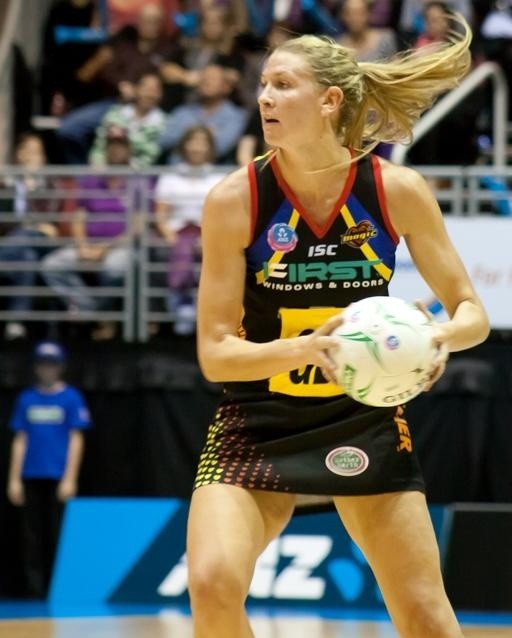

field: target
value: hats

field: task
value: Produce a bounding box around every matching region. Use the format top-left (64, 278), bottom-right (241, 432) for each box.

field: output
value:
top-left (106, 125), bottom-right (129, 141)
top-left (34, 342), bottom-right (64, 364)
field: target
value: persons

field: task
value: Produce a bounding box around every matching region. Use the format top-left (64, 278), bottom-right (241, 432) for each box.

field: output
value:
top-left (0, 1), bottom-right (511, 345)
top-left (6, 341), bottom-right (92, 601)
top-left (187, 11), bottom-right (493, 638)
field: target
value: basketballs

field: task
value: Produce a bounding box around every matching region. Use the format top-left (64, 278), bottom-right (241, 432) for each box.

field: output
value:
top-left (325, 296), bottom-right (440, 408)
